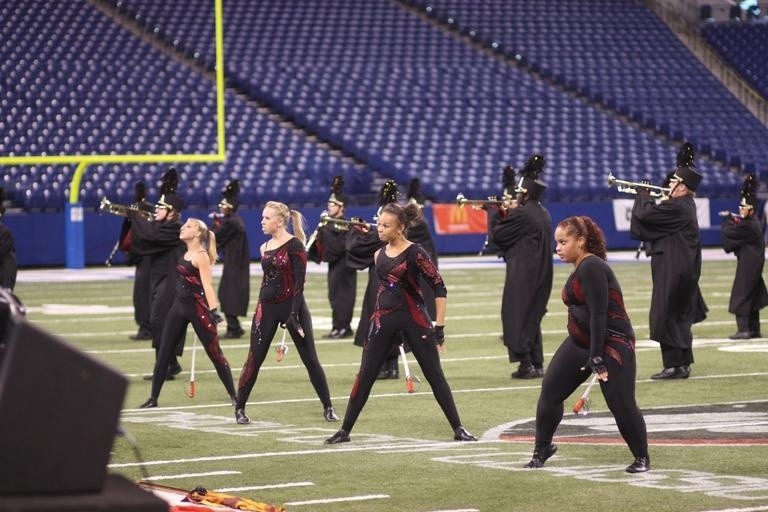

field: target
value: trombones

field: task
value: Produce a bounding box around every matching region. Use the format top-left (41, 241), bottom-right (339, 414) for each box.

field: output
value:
top-left (95, 195), bottom-right (157, 223)
top-left (605, 171), bottom-right (673, 196)
top-left (318, 208), bottom-right (377, 233)
top-left (454, 191), bottom-right (518, 210)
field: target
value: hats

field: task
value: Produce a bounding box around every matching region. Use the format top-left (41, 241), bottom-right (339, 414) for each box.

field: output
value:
top-left (501, 166), bottom-right (516, 201)
top-left (371, 180), bottom-right (400, 220)
top-left (327, 176), bottom-right (349, 218)
top-left (133, 179), bottom-right (155, 215)
top-left (737, 173), bottom-right (757, 217)
top-left (218, 179), bottom-right (241, 217)
top-left (153, 166), bottom-right (184, 222)
top-left (669, 142), bottom-right (703, 195)
top-left (405, 177), bottom-right (426, 209)
top-left (514, 153), bottom-right (548, 205)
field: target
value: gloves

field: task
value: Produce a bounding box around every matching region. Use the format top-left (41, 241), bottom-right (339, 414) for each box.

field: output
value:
top-left (432, 326), bottom-right (445, 345)
top-left (287, 312), bottom-right (302, 330)
top-left (585, 356), bottom-right (607, 375)
top-left (209, 308), bottom-right (223, 325)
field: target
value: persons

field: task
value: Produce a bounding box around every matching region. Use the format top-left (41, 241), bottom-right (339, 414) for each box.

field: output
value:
top-left (1, 206), bottom-right (24, 346)
top-left (324, 202), bottom-right (478, 445)
top-left (239, 202), bottom-right (338, 423)
top-left (126, 194), bottom-right (189, 340)
top-left (525, 217), bottom-right (650, 473)
top-left (139, 218), bottom-right (237, 407)
top-left (631, 167), bottom-right (709, 380)
top-left (306, 193), bottom-right (358, 341)
top-left (722, 196), bottom-right (767, 338)
top-left (484, 179), bottom-right (553, 379)
top-left (119, 199), bottom-right (160, 338)
top-left (345, 196), bottom-right (437, 381)
top-left (210, 193), bottom-right (249, 340)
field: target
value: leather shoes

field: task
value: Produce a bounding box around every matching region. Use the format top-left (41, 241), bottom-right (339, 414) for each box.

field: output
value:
top-left (332, 327), bottom-right (355, 338)
top-left (524, 444), bottom-right (559, 469)
top-left (229, 393), bottom-right (237, 406)
top-left (128, 326), bottom-right (151, 340)
top-left (511, 364), bottom-right (538, 379)
top-left (626, 455), bottom-right (651, 474)
top-left (323, 407), bottom-right (340, 422)
top-left (143, 374), bottom-right (175, 381)
top-left (650, 366), bottom-right (687, 380)
top-left (728, 331), bottom-right (752, 339)
top-left (535, 368), bottom-right (544, 377)
top-left (139, 398), bottom-right (158, 408)
top-left (453, 426), bottom-right (477, 442)
top-left (375, 367), bottom-right (401, 379)
top-left (166, 359), bottom-right (182, 375)
top-left (682, 366), bottom-right (691, 379)
top-left (220, 329), bottom-right (241, 339)
top-left (324, 328), bottom-right (338, 339)
top-left (324, 429), bottom-right (350, 444)
top-left (240, 327), bottom-right (245, 335)
top-left (389, 368), bottom-right (398, 379)
top-left (235, 408), bottom-right (251, 424)
top-left (752, 330), bottom-right (761, 338)
